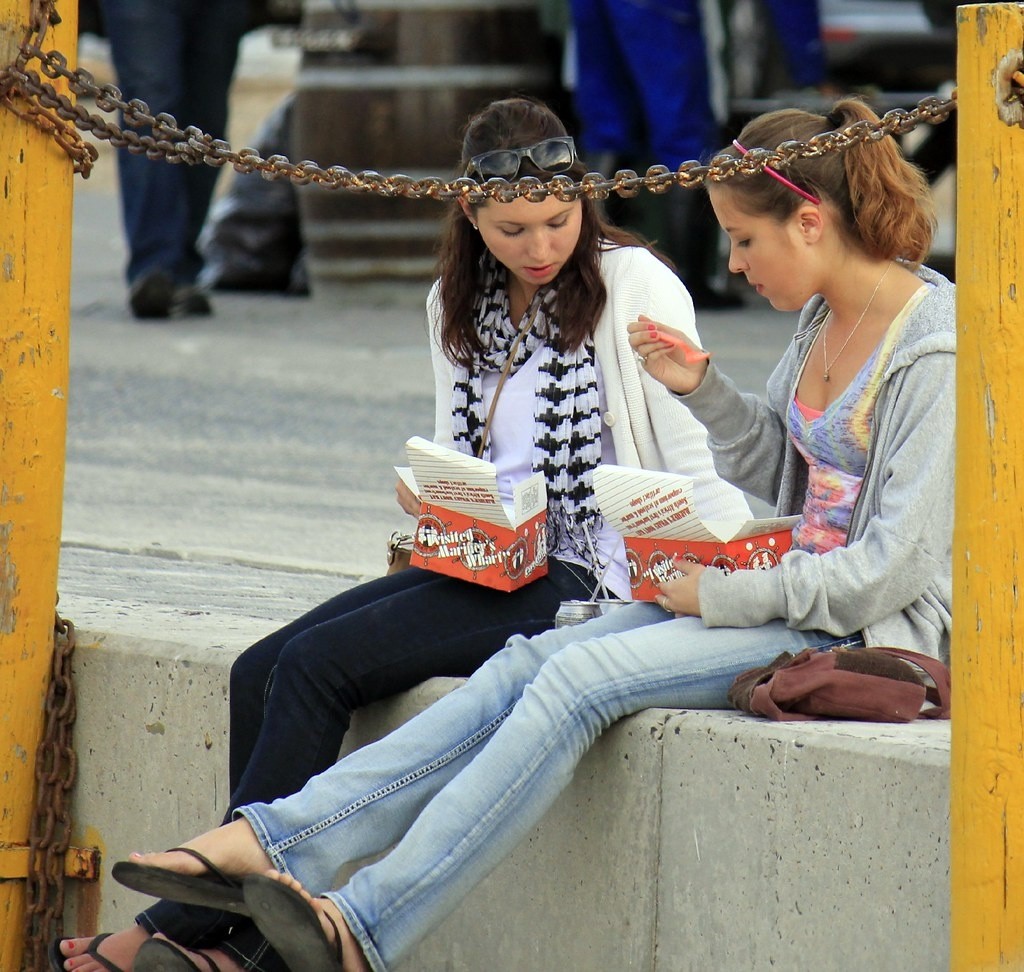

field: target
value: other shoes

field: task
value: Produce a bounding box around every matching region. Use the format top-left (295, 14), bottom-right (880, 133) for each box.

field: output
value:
top-left (130, 260), bottom-right (180, 319)
top-left (172, 282), bottom-right (215, 320)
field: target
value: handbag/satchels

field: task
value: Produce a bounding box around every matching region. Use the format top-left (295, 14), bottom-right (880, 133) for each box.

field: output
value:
top-left (732, 633), bottom-right (926, 727)
top-left (381, 528), bottom-right (413, 582)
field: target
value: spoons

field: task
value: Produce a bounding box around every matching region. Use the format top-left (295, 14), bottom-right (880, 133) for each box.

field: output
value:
top-left (657, 332), bottom-right (715, 363)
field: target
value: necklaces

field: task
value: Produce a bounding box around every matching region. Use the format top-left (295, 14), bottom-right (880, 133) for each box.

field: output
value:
top-left (823, 260), bottom-right (895, 382)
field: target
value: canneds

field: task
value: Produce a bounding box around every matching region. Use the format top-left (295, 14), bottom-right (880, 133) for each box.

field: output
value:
top-left (555, 600), bottom-right (602, 629)
top-left (595, 599), bottom-right (633, 615)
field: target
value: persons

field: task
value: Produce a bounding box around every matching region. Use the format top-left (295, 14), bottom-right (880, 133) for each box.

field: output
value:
top-left (561, 0), bottom-right (847, 313)
top-left (77, 0), bottom-right (305, 317)
top-left (111, 95), bottom-right (957, 971)
top-left (44, 98), bottom-right (721, 972)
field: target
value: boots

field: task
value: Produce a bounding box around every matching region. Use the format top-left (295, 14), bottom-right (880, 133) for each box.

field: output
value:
top-left (580, 143), bottom-right (639, 238)
top-left (660, 174), bottom-right (750, 316)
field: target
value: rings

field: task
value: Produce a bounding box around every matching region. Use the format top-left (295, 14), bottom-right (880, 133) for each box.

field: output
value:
top-left (661, 596), bottom-right (673, 615)
top-left (635, 350), bottom-right (648, 365)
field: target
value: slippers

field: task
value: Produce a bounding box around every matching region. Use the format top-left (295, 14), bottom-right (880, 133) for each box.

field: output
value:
top-left (241, 869), bottom-right (355, 972)
top-left (128, 931), bottom-right (224, 972)
top-left (110, 848), bottom-right (252, 924)
top-left (48, 928), bottom-right (122, 972)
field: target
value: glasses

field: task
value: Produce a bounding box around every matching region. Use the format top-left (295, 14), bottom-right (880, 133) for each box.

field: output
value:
top-left (461, 136), bottom-right (581, 189)
